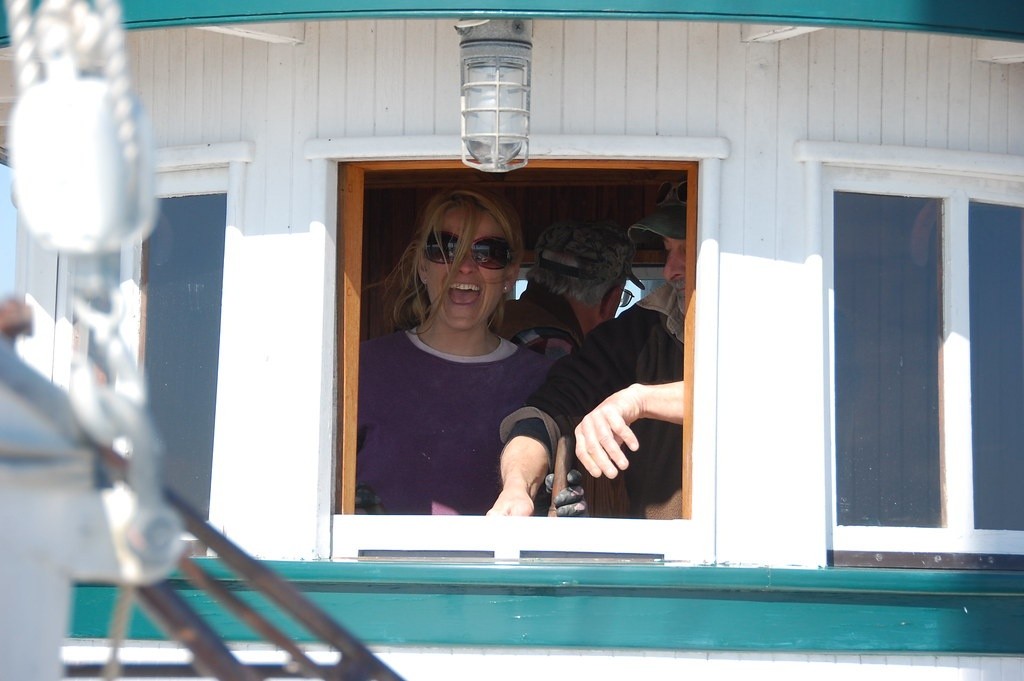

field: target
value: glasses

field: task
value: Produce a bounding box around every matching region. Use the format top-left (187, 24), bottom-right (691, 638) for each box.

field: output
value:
top-left (423, 231), bottom-right (512, 270)
top-left (656, 181), bottom-right (687, 206)
top-left (616, 287), bottom-right (635, 307)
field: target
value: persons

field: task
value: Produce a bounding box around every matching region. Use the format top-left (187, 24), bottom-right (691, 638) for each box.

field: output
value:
top-left (354, 188), bottom-right (588, 517)
top-left (487, 218), bottom-right (645, 518)
top-left (485, 180), bottom-right (687, 519)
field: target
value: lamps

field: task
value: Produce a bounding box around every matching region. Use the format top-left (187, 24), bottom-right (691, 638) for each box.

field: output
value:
top-left (456, 17), bottom-right (530, 174)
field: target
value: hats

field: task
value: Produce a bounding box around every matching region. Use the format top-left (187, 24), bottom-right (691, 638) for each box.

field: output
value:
top-left (533, 221), bottom-right (646, 290)
top-left (628, 171), bottom-right (687, 240)
